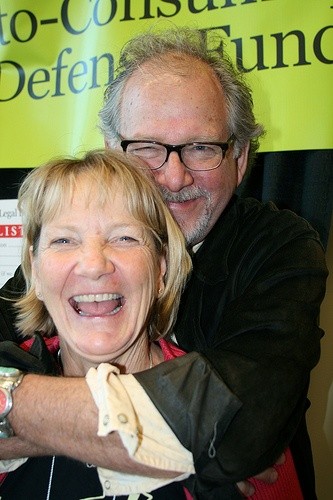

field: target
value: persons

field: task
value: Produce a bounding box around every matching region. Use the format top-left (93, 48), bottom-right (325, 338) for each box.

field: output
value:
top-left (1, 23), bottom-right (332, 500)
top-left (0, 152), bottom-right (287, 500)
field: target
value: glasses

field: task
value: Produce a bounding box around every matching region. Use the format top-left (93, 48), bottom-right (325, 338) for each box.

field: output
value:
top-left (115, 132), bottom-right (235, 171)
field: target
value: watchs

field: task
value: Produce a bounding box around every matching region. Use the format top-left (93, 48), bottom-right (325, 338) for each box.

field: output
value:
top-left (0, 363), bottom-right (24, 440)
